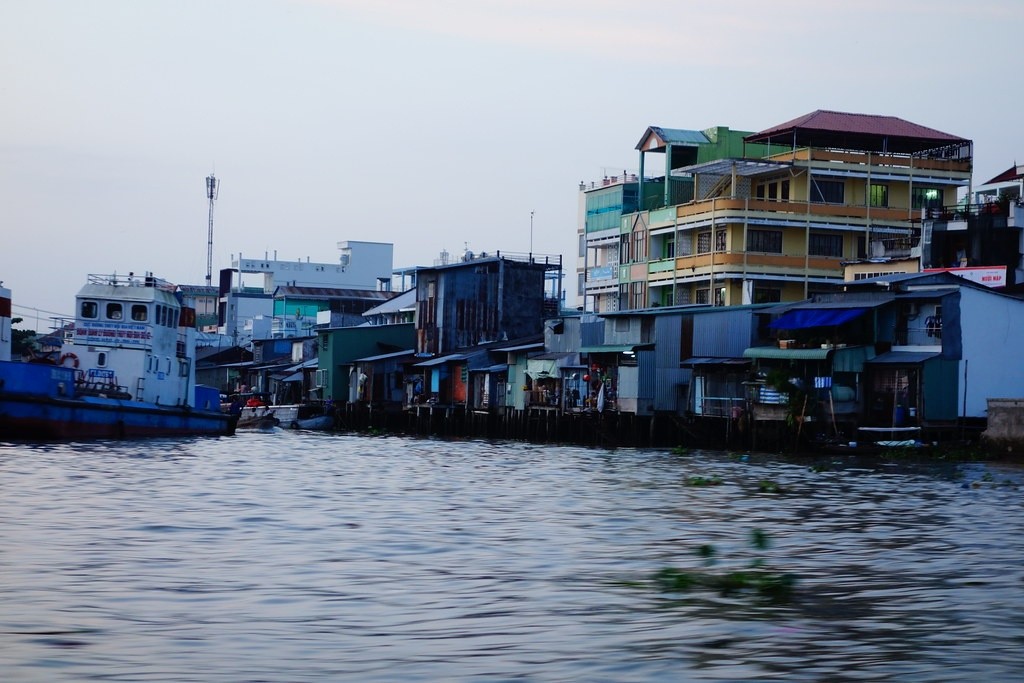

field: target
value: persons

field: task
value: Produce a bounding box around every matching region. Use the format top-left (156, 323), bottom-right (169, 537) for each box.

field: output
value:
top-left (128, 272), bottom-right (136, 287)
top-left (325, 395), bottom-right (333, 414)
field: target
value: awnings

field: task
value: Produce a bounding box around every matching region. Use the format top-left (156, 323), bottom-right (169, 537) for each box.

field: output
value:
top-left (865, 351), bottom-right (940, 363)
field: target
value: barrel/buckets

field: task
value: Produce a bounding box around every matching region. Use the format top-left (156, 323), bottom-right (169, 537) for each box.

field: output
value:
top-left (895, 406), bottom-right (904, 425)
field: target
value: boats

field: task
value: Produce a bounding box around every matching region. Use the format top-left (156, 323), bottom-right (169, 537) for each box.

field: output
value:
top-left (0, 264), bottom-right (252, 437)
top-left (240, 401), bottom-right (299, 433)
top-left (295, 413), bottom-right (336, 431)
top-left (807, 436), bottom-right (940, 463)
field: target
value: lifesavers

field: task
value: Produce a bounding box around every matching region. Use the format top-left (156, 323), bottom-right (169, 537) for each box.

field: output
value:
top-left (290, 421), bottom-right (300, 430)
top-left (60, 352), bottom-right (80, 369)
